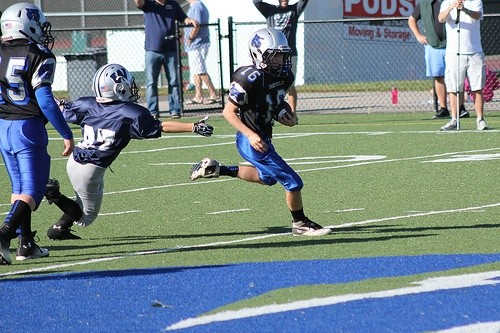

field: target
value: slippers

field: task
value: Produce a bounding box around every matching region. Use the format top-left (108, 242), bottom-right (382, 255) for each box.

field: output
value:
top-left (207, 97), bottom-right (220, 104)
top-left (185, 99), bottom-right (203, 105)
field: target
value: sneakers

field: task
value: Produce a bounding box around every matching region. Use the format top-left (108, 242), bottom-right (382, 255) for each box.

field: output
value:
top-left (432, 107), bottom-right (450, 118)
top-left (42, 178), bottom-right (60, 204)
top-left (292, 217), bottom-right (332, 236)
top-left (189, 157), bottom-right (222, 180)
top-left (440, 120), bottom-right (460, 131)
top-left (47, 226), bottom-right (81, 240)
top-left (459, 105), bottom-right (468, 117)
top-left (477, 119), bottom-right (488, 130)
top-left (16, 230), bottom-right (49, 260)
top-left (0, 222), bottom-right (19, 265)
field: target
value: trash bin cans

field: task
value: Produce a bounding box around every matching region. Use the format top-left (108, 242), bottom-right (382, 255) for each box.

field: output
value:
top-left (62, 50), bottom-right (108, 102)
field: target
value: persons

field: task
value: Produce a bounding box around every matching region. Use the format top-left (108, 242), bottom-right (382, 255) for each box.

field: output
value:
top-left (408, 0), bottom-right (470, 119)
top-left (0, 1), bottom-right (74, 261)
top-left (188, 27), bottom-right (333, 238)
top-left (440, 0), bottom-right (489, 131)
top-left (134, 0), bottom-right (199, 120)
top-left (43, 63), bottom-right (213, 240)
top-left (253, 0), bottom-right (309, 125)
top-left (184, 0), bottom-right (222, 105)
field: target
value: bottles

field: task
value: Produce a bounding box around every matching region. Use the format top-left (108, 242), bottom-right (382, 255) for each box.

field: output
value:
top-left (392, 87), bottom-right (398, 104)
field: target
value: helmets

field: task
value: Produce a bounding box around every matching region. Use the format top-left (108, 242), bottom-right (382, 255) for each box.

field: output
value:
top-left (92, 63), bottom-right (136, 103)
top-left (0, 2), bottom-right (47, 43)
top-left (248, 28), bottom-right (290, 69)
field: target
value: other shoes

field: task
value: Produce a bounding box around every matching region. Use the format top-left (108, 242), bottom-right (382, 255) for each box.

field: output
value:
top-left (153, 115), bottom-right (160, 118)
top-left (172, 115), bottom-right (181, 119)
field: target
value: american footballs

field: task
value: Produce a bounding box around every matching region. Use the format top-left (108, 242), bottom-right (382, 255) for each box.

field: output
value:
top-left (273, 100), bottom-right (292, 122)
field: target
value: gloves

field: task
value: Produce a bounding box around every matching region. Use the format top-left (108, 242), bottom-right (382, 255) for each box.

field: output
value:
top-left (192, 115), bottom-right (214, 137)
